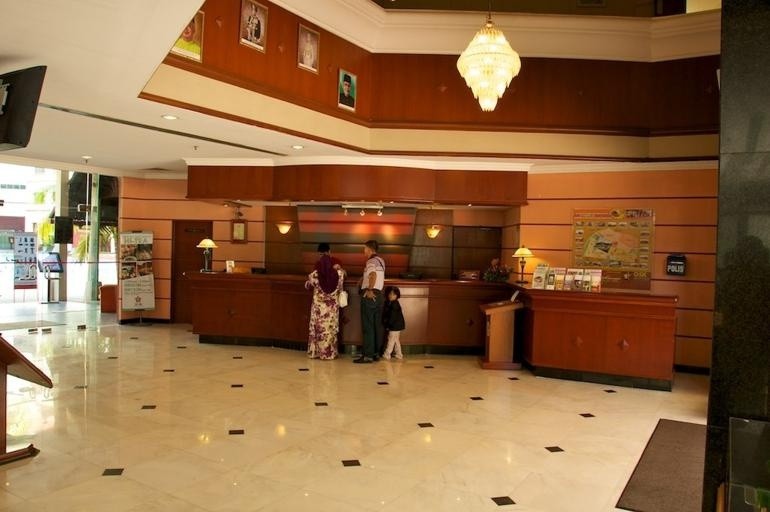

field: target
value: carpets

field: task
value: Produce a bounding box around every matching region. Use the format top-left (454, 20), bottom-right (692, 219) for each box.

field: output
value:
top-left (614, 417), bottom-right (707, 512)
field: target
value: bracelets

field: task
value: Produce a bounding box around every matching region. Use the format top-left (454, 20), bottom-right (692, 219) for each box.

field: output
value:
top-left (338, 266), bottom-right (342, 270)
top-left (367, 288), bottom-right (373, 291)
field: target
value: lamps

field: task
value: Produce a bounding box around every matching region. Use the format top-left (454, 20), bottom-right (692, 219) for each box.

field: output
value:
top-left (511, 245), bottom-right (536, 284)
top-left (455, 1), bottom-right (523, 114)
top-left (196, 236), bottom-right (218, 272)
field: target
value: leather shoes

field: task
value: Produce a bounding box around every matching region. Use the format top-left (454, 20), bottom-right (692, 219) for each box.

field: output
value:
top-left (353, 355), bottom-right (373, 363)
top-left (373, 353), bottom-right (380, 361)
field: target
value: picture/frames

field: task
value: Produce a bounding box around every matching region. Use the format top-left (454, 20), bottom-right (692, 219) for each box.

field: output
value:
top-left (230, 219), bottom-right (248, 244)
top-left (168, 10), bottom-right (205, 65)
top-left (297, 22), bottom-right (321, 76)
top-left (239, 0), bottom-right (269, 55)
top-left (338, 67), bottom-right (358, 114)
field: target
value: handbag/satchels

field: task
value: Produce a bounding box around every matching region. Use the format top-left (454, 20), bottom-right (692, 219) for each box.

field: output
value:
top-left (337, 290), bottom-right (348, 308)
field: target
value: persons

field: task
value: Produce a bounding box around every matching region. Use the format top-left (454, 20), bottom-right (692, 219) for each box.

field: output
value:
top-left (379, 286), bottom-right (406, 359)
top-left (311, 242), bottom-right (347, 278)
top-left (353, 240), bottom-right (386, 363)
top-left (304, 255), bottom-right (344, 361)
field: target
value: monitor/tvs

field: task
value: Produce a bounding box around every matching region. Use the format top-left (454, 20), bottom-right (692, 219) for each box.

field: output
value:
top-left (0, 65), bottom-right (47, 152)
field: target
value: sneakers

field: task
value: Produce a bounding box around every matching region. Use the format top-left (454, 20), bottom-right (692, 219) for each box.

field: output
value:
top-left (392, 355), bottom-right (402, 360)
